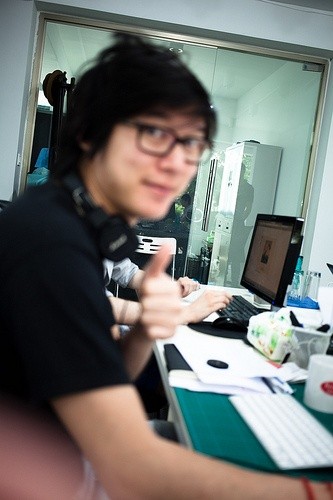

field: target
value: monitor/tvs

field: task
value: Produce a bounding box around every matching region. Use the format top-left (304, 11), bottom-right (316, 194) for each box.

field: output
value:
top-left (240, 214), bottom-right (305, 308)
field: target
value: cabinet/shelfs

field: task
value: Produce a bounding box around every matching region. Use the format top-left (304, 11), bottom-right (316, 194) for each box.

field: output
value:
top-left (207, 142), bottom-right (283, 286)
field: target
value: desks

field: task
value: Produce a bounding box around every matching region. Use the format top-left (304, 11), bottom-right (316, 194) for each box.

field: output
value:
top-left (154, 284), bottom-right (333, 479)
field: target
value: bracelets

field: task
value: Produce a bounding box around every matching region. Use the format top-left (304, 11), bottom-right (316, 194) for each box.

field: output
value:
top-left (301, 477), bottom-right (315, 500)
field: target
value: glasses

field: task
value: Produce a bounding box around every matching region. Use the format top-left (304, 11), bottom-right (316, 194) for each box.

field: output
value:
top-left (119, 119), bottom-right (214, 166)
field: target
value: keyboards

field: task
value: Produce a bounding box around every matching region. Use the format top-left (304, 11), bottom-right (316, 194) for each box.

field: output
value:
top-left (216, 295), bottom-right (261, 325)
top-left (228, 393), bottom-right (333, 470)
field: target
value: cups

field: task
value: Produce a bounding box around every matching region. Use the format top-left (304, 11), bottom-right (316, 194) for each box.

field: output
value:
top-left (284, 325), bottom-right (331, 384)
top-left (304, 354), bottom-right (333, 414)
top-left (286, 268), bottom-right (321, 309)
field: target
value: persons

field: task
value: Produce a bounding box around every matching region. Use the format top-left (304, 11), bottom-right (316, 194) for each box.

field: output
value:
top-left (0, 32), bottom-right (333, 500)
top-left (103, 254), bottom-right (233, 326)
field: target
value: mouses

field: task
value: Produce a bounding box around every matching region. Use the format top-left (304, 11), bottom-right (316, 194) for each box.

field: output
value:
top-left (212, 316), bottom-right (239, 330)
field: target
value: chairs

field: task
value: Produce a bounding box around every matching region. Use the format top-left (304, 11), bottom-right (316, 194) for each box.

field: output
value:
top-left (116, 235), bottom-right (178, 297)
